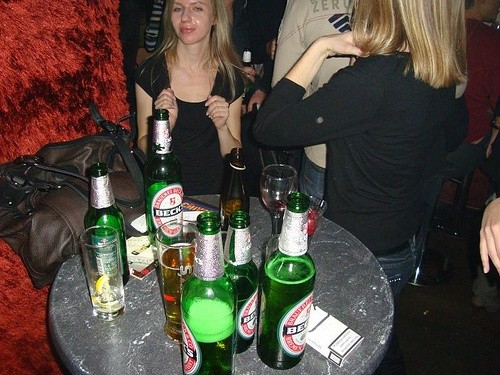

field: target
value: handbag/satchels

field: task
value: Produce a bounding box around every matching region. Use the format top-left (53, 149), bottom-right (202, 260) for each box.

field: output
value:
top-left (0, 101), bottom-right (154, 288)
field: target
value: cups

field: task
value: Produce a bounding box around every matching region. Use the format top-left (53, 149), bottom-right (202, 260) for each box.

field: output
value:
top-left (266, 235), bottom-right (279, 260)
top-left (308, 195), bottom-right (328, 244)
top-left (155, 220), bottom-right (201, 343)
top-left (79, 225), bottom-right (125, 322)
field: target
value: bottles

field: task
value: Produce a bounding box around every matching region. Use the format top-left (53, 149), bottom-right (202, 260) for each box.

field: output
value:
top-left (219, 147), bottom-right (250, 231)
top-left (222, 210), bottom-right (260, 354)
top-left (83, 163), bottom-right (129, 285)
top-left (145, 107), bottom-right (185, 244)
top-left (256, 192), bottom-right (317, 369)
top-left (241, 47), bottom-right (254, 94)
top-left (180, 212), bottom-right (238, 375)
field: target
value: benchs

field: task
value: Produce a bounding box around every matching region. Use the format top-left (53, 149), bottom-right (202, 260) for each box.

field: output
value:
top-left (0, 0), bottom-right (132, 375)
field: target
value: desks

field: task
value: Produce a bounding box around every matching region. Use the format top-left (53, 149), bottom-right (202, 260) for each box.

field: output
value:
top-left (45, 193), bottom-right (396, 375)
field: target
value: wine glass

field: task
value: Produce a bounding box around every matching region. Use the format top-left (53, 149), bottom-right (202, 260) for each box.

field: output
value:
top-left (260, 164), bottom-right (299, 235)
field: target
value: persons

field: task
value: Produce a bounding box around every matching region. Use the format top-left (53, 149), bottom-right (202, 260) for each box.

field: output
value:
top-left (460, 0), bottom-right (500, 275)
top-left (134, 0), bottom-right (288, 196)
top-left (271, 0), bottom-right (356, 205)
top-left (253, 0), bottom-right (465, 301)
